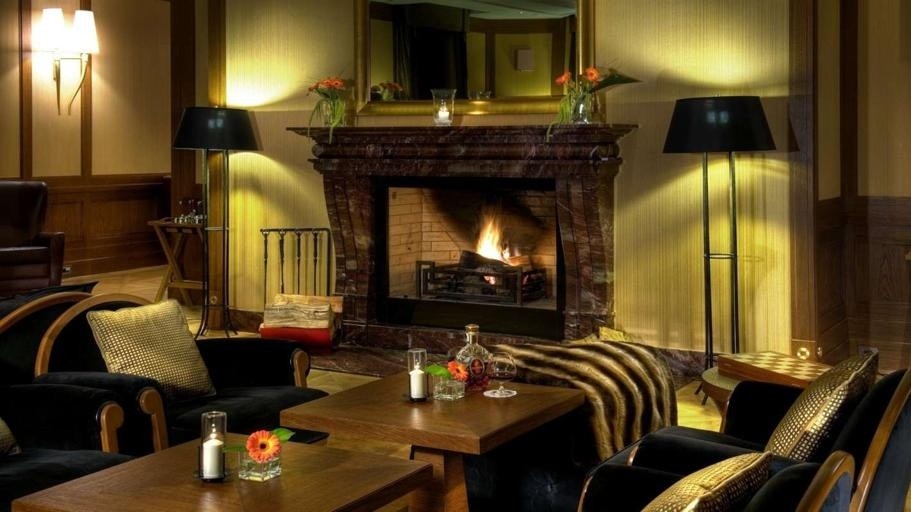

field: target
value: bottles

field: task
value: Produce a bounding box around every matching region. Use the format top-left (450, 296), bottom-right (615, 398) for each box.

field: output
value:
top-left (455, 322), bottom-right (494, 394)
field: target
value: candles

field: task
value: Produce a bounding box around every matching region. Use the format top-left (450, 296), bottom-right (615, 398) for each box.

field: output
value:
top-left (408, 357), bottom-right (428, 400)
top-left (431, 100), bottom-right (455, 126)
top-left (199, 420), bottom-right (227, 479)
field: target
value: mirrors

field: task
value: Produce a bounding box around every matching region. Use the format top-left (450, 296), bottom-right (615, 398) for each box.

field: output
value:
top-left (353, 1), bottom-right (593, 113)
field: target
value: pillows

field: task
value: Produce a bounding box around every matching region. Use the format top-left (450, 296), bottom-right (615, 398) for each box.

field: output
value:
top-left (84, 297), bottom-right (220, 400)
top-left (764, 344), bottom-right (882, 466)
top-left (0, 411), bottom-right (25, 463)
top-left (638, 446), bottom-right (775, 511)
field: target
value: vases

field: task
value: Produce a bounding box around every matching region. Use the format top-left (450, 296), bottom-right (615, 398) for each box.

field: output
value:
top-left (319, 100), bottom-right (348, 129)
top-left (562, 89), bottom-right (597, 126)
top-left (236, 448), bottom-right (288, 485)
top-left (432, 378), bottom-right (467, 401)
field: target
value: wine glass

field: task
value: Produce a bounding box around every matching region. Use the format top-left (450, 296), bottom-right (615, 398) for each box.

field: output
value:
top-left (487, 351), bottom-right (516, 395)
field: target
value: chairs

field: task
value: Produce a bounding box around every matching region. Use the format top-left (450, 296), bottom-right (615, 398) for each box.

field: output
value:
top-left (0, 289), bottom-right (95, 378)
top-left (34, 291), bottom-right (335, 458)
top-left (1, 177), bottom-right (69, 295)
top-left (0, 378), bottom-right (135, 512)
top-left (650, 365), bottom-right (911, 512)
top-left (578, 448), bottom-right (864, 512)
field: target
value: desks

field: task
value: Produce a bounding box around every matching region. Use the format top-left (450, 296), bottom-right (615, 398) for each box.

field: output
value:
top-left (277, 363), bottom-right (590, 511)
top-left (8, 428), bottom-right (437, 512)
top-left (150, 210), bottom-right (211, 309)
top-left (700, 365), bottom-right (744, 415)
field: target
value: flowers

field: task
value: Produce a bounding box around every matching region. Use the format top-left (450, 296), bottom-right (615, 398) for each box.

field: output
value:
top-left (309, 75), bottom-right (351, 129)
top-left (554, 64), bottom-right (604, 93)
top-left (245, 425), bottom-right (297, 464)
top-left (367, 77), bottom-right (404, 106)
top-left (427, 359), bottom-right (470, 381)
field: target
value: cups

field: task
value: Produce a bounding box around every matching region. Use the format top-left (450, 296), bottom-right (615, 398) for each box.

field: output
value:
top-left (202, 409), bottom-right (226, 480)
top-left (409, 347), bottom-right (428, 401)
top-left (430, 87), bottom-right (456, 126)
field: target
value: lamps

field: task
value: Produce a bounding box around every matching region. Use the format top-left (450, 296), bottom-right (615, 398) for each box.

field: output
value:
top-left (659, 94), bottom-right (779, 407)
top-left (169, 105), bottom-right (264, 341)
top-left (31, 2), bottom-right (103, 118)
